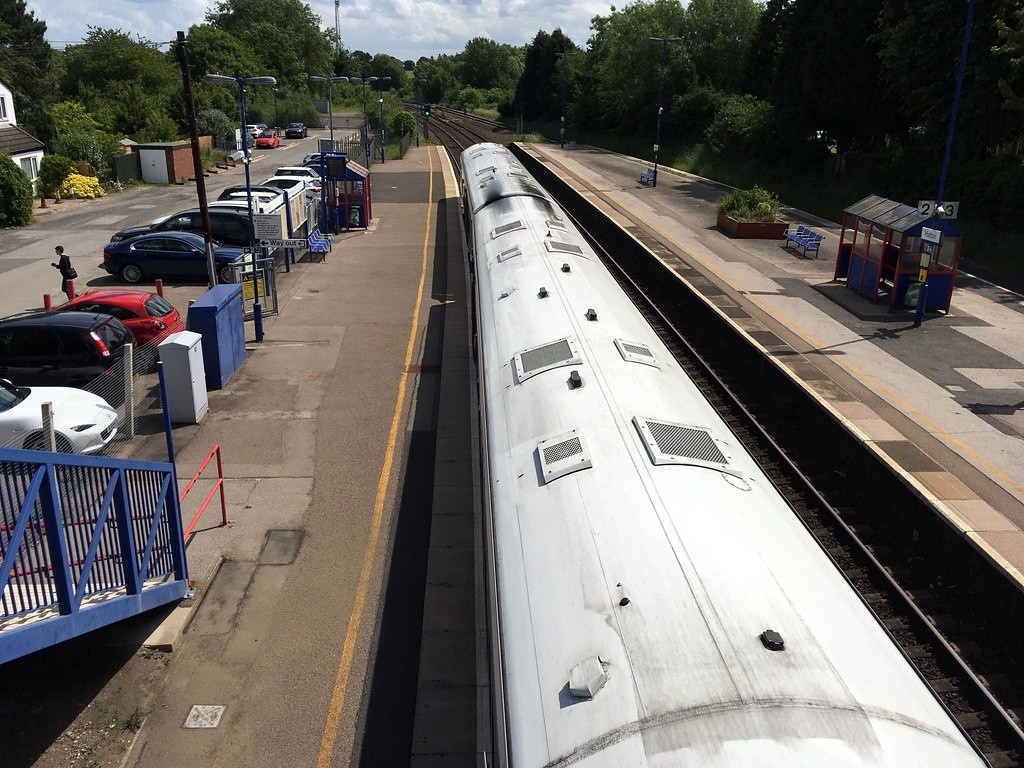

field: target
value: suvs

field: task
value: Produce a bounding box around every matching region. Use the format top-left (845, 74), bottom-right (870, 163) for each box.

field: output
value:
top-left (0, 311), bottom-right (138, 407)
top-left (274, 165), bottom-right (321, 183)
top-left (110, 205), bottom-right (260, 249)
top-left (246, 125), bottom-right (263, 138)
top-left (209, 185), bottom-right (285, 210)
top-left (254, 175), bottom-right (322, 202)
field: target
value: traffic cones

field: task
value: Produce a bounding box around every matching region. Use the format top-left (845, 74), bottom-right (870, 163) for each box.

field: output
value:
top-left (66, 184), bottom-right (77, 200)
top-left (38, 192), bottom-right (49, 208)
top-left (54, 188), bottom-right (64, 203)
top-left (114, 177), bottom-right (122, 191)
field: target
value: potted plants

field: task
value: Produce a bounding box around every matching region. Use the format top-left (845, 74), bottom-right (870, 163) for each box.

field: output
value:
top-left (715, 183), bottom-right (789, 240)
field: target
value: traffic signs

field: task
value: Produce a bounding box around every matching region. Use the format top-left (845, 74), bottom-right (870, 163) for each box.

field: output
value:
top-left (261, 240), bottom-right (306, 248)
top-left (244, 246), bottom-right (261, 253)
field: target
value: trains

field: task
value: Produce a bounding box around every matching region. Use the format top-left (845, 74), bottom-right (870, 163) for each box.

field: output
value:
top-left (459, 143), bottom-right (990, 768)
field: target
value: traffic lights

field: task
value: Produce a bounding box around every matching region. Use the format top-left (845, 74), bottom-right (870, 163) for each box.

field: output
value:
top-left (424, 104), bottom-right (431, 116)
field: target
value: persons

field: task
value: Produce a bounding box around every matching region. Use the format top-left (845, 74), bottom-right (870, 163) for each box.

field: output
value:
top-left (53, 246), bottom-right (79, 300)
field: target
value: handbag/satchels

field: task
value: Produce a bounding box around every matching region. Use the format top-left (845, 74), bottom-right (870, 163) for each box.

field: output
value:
top-left (63, 268), bottom-right (78, 279)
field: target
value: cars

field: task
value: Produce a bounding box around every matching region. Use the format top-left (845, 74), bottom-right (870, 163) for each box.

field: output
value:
top-left (0, 379), bottom-right (119, 454)
top-left (256, 132), bottom-right (280, 149)
top-left (295, 153), bottom-right (329, 176)
top-left (50, 289), bottom-right (185, 373)
top-left (285, 123), bottom-right (307, 139)
top-left (102, 231), bottom-right (245, 284)
top-left (247, 133), bottom-right (255, 149)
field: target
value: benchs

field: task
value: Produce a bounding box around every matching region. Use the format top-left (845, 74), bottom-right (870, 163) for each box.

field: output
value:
top-left (640, 168), bottom-right (653, 184)
top-left (783, 225), bottom-right (826, 258)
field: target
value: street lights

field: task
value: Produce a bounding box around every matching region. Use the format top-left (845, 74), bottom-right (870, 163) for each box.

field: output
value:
top-left (350, 77), bottom-right (377, 170)
top-left (377, 77), bottom-right (391, 122)
top-left (648, 36), bottom-right (682, 185)
top-left (206, 73), bottom-right (277, 342)
top-left (309, 76), bottom-right (349, 234)
top-left (555, 52), bottom-right (575, 149)
